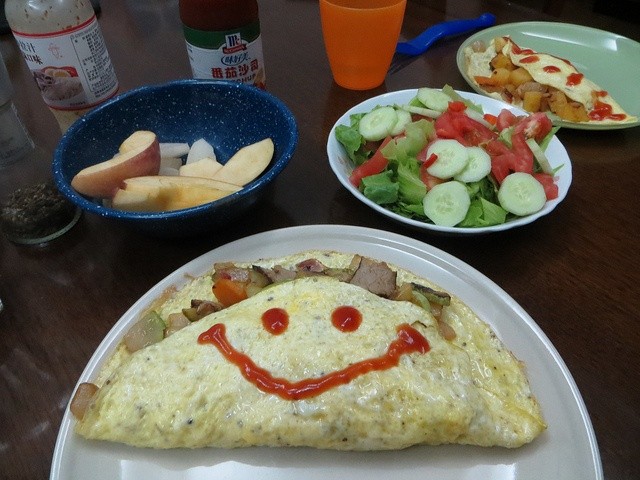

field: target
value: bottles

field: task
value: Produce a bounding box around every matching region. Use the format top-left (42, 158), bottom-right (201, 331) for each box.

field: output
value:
top-left (178, 0), bottom-right (267, 91)
top-left (5, 0), bottom-right (121, 137)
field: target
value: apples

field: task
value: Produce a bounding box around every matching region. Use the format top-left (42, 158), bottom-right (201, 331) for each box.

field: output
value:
top-left (111, 174), bottom-right (246, 212)
top-left (70, 129), bottom-right (161, 199)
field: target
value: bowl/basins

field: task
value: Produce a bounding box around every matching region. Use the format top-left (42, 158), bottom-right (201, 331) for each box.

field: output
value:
top-left (50, 78), bottom-right (299, 235)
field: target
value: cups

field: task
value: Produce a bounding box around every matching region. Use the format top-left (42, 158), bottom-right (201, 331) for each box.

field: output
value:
top-left (319, 1), bottom-right (407, 91)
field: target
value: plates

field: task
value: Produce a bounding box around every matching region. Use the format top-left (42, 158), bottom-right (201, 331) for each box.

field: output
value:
top-left (47, 223), bottom-right (605, 480)
top-left (327, 87), bottom-right (574, 234)
top-left (455, 20), bottom-right (640, 131)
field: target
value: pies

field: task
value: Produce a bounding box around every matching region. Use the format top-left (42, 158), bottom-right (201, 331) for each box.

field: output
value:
top-left (463, 32), bottom-right (638, 127)
top-left (67, 249), bottom-right (547, 453)
